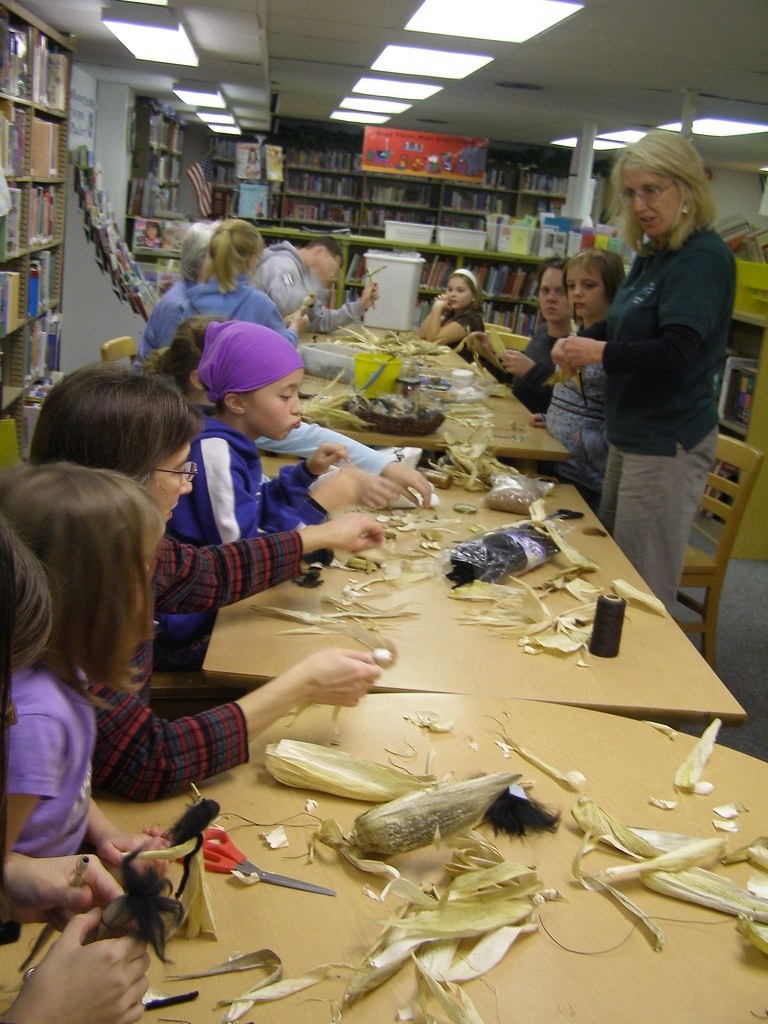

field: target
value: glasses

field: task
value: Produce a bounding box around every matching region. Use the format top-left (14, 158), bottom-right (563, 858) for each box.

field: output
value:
top-left (618, 182), bottom-right (675, 206)
top-left (154, 461), bottom-right (198, 481)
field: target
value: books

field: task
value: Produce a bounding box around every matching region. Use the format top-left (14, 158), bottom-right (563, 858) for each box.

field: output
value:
top-left (0, 28), bottom-right (67, 466)
top-left (73, 144), bottom-right (161, 321)
top-left (713, 212), bottom-right (768, 264)
top-left (126, 113), bottom-right (186, 218)
top-left (615, 254), bottom-right (621, 261)
top-left (486, 213), bottom-right (599, 251)
top-left (281, 148), bottom-right (566, 230)
top-left (416, 255), bottom-right (545, 337)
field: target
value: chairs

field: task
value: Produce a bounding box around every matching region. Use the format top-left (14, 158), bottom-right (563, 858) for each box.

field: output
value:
top-left (675, 432), bottom-right (766, 670)
top-left (98, 335), bottom-right (137, 368)
top-left (483, 322), bottom-right (513, 334)
top-left (485, 331), bottom-right (532, 354)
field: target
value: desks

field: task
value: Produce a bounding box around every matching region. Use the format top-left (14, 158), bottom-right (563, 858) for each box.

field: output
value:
top-left (201, 456), bottom-right (747, 727)
top-left (295, 324), bottom-right (569, 477)
top-left (1, 693), bottom-right (767, 1024)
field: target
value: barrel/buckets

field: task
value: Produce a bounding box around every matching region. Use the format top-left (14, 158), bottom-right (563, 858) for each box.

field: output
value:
top-left (354, 353), bottom-right (402, 395)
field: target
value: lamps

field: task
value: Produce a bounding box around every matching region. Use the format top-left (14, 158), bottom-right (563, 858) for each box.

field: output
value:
top-left (171, 83), bottom-right (242, 136)
top-left (99, 5), bottom-right (201, 69)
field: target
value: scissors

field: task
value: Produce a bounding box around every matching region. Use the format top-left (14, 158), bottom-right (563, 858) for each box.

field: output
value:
top-left (549, 509), bottom-right (584, 521)
top-left (201, 829), bottom-right (337, 899)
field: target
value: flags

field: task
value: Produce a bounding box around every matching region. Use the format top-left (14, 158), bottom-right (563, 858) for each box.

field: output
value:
top-left (188, 144), bottom-right (220, 217)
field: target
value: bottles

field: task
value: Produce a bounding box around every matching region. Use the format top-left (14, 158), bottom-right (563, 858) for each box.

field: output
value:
top-left (396, 377), bottom-right (420, 402)
top-left (401, 357), bottom-right (419, 377)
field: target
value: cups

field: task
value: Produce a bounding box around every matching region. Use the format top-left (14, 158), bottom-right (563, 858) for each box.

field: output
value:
top-left (452, 370), bottom-right (472, 389)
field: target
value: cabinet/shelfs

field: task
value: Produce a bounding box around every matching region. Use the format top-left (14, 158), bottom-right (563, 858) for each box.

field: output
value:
top-left (0, 1), bottom-right (80, 471)
top-left (128, 95), bottom-right (768, 559)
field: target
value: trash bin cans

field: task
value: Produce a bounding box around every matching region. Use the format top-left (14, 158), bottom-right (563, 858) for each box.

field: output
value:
top-left (362, 253), bottom-right (426, 331)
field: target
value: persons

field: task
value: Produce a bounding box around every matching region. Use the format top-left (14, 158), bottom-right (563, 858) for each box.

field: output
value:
top-left (138, 222), bottom-right (161, 249)
top-left (135, 219), bottom-right (380, 373)
top-left (550, 131), bottom-right (737, 617)
top-left (467, 249), bottom-right (625, 513)
top-left (0, 466), bottom-right (166, 1024)
top-left (243, 147), bottom-right (260, 180)
top-left (420, 267), bottom-right (484, 365)
top-left (30, 313), bottom-right (432, 802)
